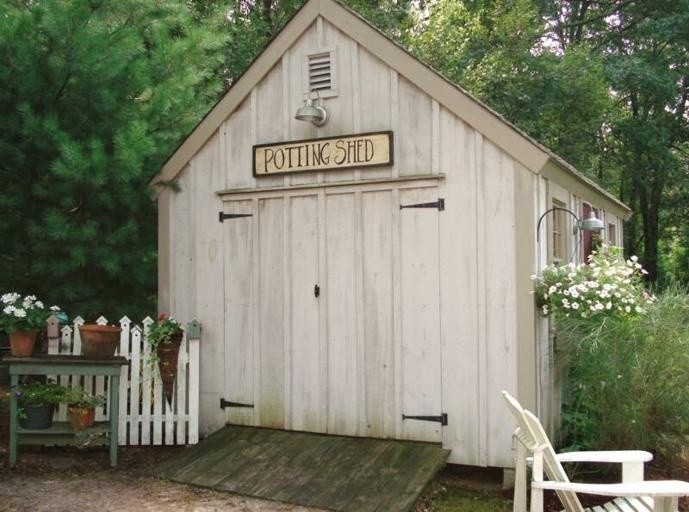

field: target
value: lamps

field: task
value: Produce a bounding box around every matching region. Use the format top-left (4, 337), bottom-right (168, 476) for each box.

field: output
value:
top-left (295, 90), bottom-right (330, 127)
top-left (576, 199), bottom-right (604, 237)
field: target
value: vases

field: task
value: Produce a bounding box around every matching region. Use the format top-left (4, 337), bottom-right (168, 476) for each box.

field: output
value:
top-left (78, 326), bottom-right (118, 360)
top-left (7, 330), bottom-right (36, 358)
top-left (20, 401), bottom-right (95, 429)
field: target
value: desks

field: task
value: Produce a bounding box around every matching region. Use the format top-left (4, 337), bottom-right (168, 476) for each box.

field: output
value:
top-left (3, 355), bottom-right (127, 466)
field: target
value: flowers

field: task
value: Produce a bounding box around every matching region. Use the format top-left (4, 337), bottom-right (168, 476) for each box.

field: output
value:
top-left (1, 292), bottom-right (50, 333)
top-left (145, 312), bottom-right (182, 364)
top-left (9, 377), bottom-right (107, 420)
top-left (532, 241), bottom-right (656, 355)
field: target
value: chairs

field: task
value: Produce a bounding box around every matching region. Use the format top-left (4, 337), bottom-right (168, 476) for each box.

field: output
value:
top-left (496, 388), bottom-right (688, 512)
